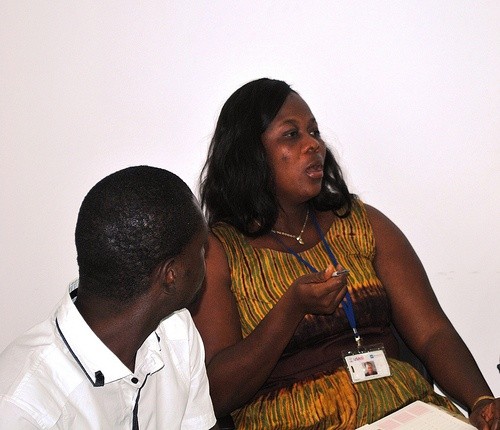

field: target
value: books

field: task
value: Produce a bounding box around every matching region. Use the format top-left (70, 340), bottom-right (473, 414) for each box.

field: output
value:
top-left (356, 401), bottom-right (478, 430)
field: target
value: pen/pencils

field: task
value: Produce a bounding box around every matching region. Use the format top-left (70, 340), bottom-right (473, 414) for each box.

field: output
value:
top-left (332, 269), bottom-right (350, 277)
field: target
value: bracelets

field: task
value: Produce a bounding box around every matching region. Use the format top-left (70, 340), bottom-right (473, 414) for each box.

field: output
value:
top-left (471, 396), bottom-right (495, 411)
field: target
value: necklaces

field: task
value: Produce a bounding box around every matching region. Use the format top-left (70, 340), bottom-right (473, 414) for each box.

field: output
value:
top-left (249, 208), bottom-right (310, 245)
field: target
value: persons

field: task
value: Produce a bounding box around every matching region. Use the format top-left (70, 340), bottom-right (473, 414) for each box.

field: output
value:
top-left (186, 78), bottom-right (500, 430)
top-left (0, 165), bottom-right (217, 430)
top-left (364, 362), bottom-right (377, 376)
